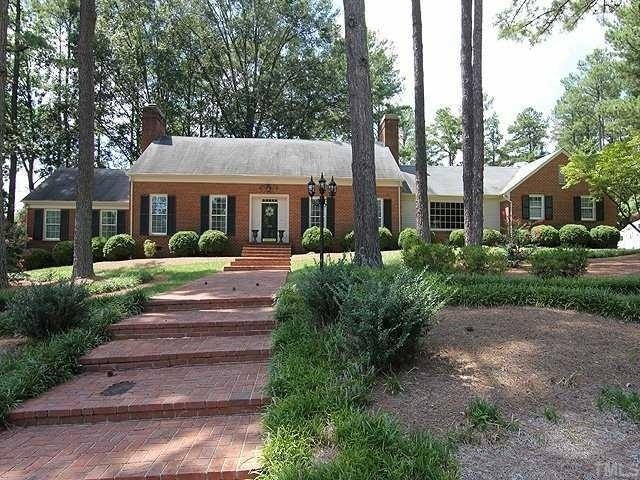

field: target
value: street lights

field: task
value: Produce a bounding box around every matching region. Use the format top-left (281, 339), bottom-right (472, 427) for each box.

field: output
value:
top-left (308, 170), bottom-right (337, 274)
